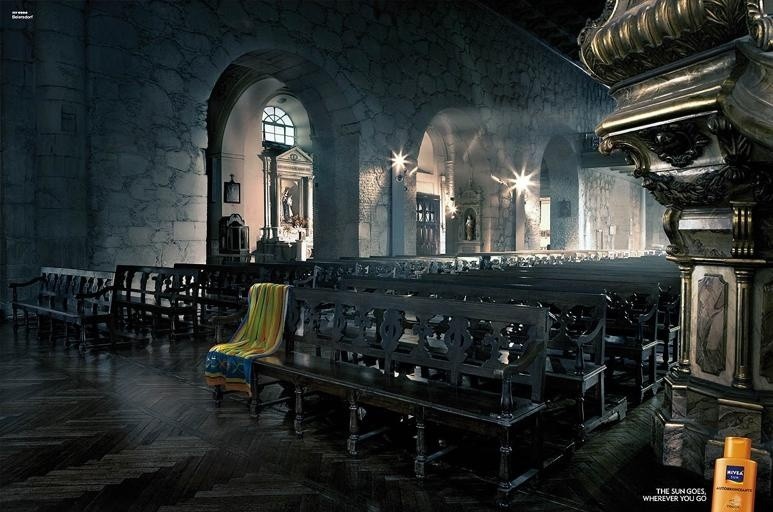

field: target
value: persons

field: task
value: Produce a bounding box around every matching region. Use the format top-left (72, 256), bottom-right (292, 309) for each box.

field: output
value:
top-left (282, 189), bottom-right (290, 220)
top-left (285, 194), bottom-right (293, 217)
top-left (464, 214), bottom-right (473, 242)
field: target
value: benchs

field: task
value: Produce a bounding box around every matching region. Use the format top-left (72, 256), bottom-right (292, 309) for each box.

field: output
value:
top-left (9, 256), bottom-right (681, 512)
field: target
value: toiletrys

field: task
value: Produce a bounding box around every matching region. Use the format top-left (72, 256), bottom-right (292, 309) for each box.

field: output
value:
top-left (711, 435), bottom-right (758, 512)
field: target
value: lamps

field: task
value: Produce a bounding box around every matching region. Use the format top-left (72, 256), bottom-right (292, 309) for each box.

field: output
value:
top-left (395, 166), bottom-right (406, 190)
